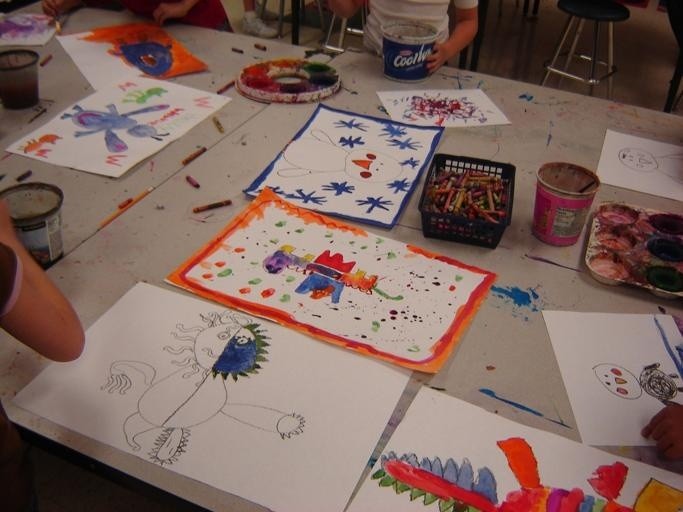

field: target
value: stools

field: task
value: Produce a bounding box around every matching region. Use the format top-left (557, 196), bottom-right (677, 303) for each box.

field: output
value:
top-left (540, 0), bottom-right (630, 101)
top-left (324, 0), bottom-right (368, 55)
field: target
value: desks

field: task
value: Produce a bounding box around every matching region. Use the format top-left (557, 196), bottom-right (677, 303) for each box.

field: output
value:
top-left (0, 0), bottom-right (334, 269)
top-left (1, 49), bottom-right (682, 512)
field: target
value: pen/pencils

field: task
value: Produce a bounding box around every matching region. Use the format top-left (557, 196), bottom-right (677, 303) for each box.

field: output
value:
top-left (182, 147), bottom-right (206, 165)
top-left (28, 109), bottom-right (47, 124)
top-left (16, 169), bottom-right (32, 181)
top-left (217, 80), bottom-right (235, 94)
top-left (213, 116), bottom-right (224, 133)
top-left (422, 167), bottom-right (506, 244)
top-left (193, 200), bottom-right (231, 212)
top-left (232, 48), bottom-right (243, 54)
top-left (40, 55), bottom-right (52, 66)
top-left (254, 44), bottom-right (266, 51)
top-left (54, 12), bottom-right (61, 34)
top-left (96, 186), bottom-right (155, 231)
top-left (185, 175), bottom-right (200, 188)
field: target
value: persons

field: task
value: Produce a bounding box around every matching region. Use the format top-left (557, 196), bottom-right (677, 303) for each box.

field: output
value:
top-left (242, 0), bottom-right (279, 38)
top-left (327, 0), bottom-right (479, 74)
top-left (0, 200), bottom-right (84, 362)
top-left (42, 0), bottom-right (237, 36)
top-left (641, 405), bottom-right (683, 459)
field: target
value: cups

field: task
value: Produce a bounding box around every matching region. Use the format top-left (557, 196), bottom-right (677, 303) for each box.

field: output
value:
top-left (533, 161), bottom-right (600, 247)
top-left (0, 182), bottom-right (64, 270)
top-left (381, 20), bottom-right (439, 84)
top-left (0, 49), bottom-right (39, 110)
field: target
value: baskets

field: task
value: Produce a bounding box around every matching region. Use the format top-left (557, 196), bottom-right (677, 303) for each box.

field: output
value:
top-left (418, 153), bottom-right (516, 249)
